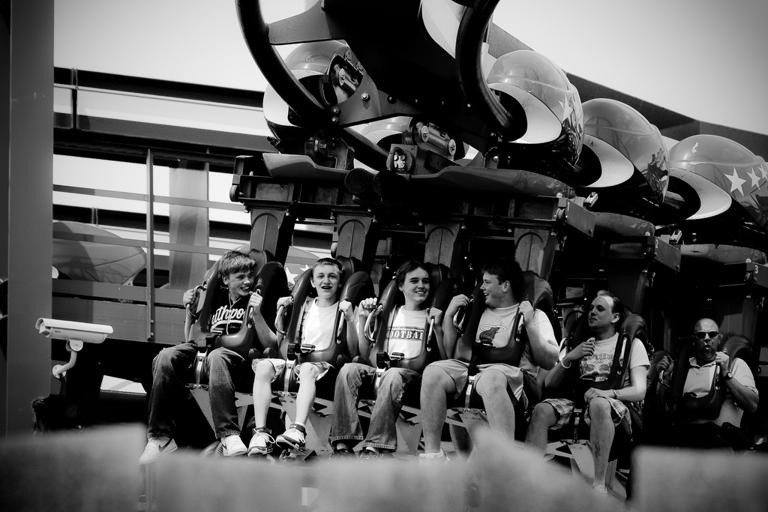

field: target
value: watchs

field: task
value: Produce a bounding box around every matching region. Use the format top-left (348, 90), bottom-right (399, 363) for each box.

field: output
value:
top-left (722, 371), bottom-right (734, 383)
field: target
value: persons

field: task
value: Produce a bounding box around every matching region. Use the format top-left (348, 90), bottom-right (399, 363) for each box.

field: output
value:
top-left (327, 260), bottom-right (445, 460)
top-left (615, 316), bottom-right (759, 484)
top-left (418, 257), bottom-right (561, 460)
top-left (138, 249), bottom-right (279, 465)
top-left (242, 256), bottom-right (361, 458)
top-left (525, 293), bottom-right (649, 495)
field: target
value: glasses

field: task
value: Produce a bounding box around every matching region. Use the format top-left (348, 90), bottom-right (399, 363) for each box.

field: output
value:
top-left (695, 331), bottom-right (720, 340)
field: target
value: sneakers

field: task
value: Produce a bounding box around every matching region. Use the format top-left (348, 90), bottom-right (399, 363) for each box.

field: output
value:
top-left (275, 423), bottom-right (307, 453)
top-left (247, 427), bottom-right (274, 457)
top-left (220, 432), bottom-right (247, 457)
top-left (137, 435), bottom-right (178, 466)
top-left (333, 446), bottom-right (383, 464)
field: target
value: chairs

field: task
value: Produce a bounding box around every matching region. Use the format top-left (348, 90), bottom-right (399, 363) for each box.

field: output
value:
top-left (366, 259), bottom-right (455, 448)
top-left (172, 247), bottom-right (286, 447)
top-left (447, 267), bottom-right (562, 445)
top-left (555, 297), bottom-right (753, 447)
top-left (276, 253), bottom-right (377, 448)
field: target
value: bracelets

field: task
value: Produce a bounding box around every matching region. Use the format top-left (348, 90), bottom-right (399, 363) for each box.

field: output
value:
top-left (610, 388), bottom-right (619, 399)
top-left (561, 356), bottom-right (572, 369)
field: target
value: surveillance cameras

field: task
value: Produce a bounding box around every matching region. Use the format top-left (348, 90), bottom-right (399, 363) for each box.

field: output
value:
top-left (36, 318), bottom-right (114, 344)
